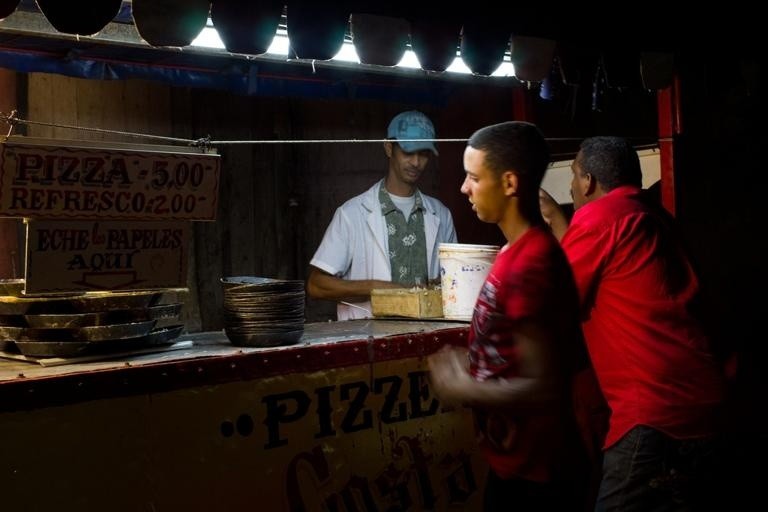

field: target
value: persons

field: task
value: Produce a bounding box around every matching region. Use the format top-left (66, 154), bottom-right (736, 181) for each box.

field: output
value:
top-left (304, 110), bottom-right (460, 323)
top-left (422, 119), bottom-right (615, 510)
top-left (530, 131), bottom-right (764, 512)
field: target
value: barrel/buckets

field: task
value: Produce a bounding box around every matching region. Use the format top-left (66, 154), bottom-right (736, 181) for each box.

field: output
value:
top-left (437, 243), bottom-right (502, 321)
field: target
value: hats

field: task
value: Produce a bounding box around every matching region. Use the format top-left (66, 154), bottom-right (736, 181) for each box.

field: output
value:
top-left (388, 109), bottom-right (440, 157)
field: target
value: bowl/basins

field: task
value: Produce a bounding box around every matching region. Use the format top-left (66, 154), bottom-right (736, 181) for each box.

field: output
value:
top-left (220, 274), bottom-right (307, 346)
